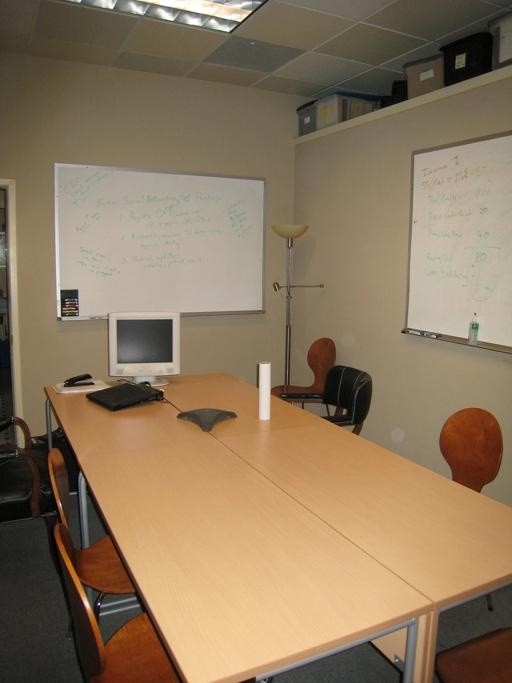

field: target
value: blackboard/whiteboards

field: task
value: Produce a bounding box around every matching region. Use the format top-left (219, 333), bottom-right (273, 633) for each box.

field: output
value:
top-left (54, 163), bottom-right (264, 321)
top-left (400, 130), bottom-right (512, 354)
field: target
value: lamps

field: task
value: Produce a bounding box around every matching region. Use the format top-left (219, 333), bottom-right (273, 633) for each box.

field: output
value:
top-left (272, 223), bottom-right (310, 386)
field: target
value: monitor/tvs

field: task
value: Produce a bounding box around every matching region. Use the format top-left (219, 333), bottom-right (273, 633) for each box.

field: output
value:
top-left (109, 312), bottom-right (181, 386)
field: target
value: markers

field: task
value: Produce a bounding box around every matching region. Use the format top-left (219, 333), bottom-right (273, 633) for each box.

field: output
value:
top-left (402, 329), bottom-right (437, 338)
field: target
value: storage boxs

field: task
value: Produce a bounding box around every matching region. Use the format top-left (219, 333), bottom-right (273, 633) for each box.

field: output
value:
top-left (295, 12), bottom-right (512, 137)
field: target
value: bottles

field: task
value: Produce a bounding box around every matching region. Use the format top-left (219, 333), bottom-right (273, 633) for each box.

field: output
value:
top-left (467, 313), bottom-right (479, 344)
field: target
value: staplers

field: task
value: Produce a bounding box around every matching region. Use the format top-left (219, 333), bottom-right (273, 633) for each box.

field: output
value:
top-left (64, 374), bottom-right (94, 387)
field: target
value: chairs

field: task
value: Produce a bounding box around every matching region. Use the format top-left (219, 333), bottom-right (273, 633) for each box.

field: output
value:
top-left (0, 415), bottom-right (73, 616)
top-left (271, 338), bottom-right (336, 416)
top-left (279, 365), bottom-right (372, 436)
